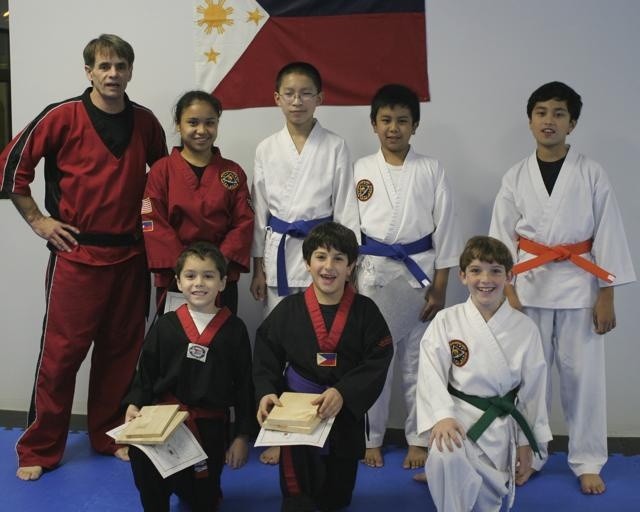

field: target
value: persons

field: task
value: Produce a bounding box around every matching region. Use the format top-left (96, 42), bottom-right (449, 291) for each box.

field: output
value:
top-left (1, 32), bottom-right (169, 481)
top-left (487, 80), bottom-right (635, 495)
top-left (141, 89), bottom-right (255, 317)
top-left (414, 235), bottom-right (555, 511)
top-left (120, 239), bottom-right (258, 512)
top-left (248, 59), bottom-right (363, 464)
top-left (353, 81), bottom-right (465, 469)
top-left (251, 222), bottom-right (395, 512)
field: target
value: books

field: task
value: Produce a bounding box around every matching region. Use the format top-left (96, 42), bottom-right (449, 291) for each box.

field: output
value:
top-left (267, 392), bottom-right (324, 426)
top-left (115, 411), bottom-right (190, 445)
top-left (263, 411), bottom-right (327, 433)
top-left (126, 404), bottom-right (180, 438)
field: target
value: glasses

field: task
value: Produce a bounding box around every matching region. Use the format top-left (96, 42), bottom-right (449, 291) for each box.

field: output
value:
top-left (277, 92), bottom-right (319, 102)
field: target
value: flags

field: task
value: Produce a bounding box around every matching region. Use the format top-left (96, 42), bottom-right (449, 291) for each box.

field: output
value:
top-left (192, 0), bottom-right (432, 109)
